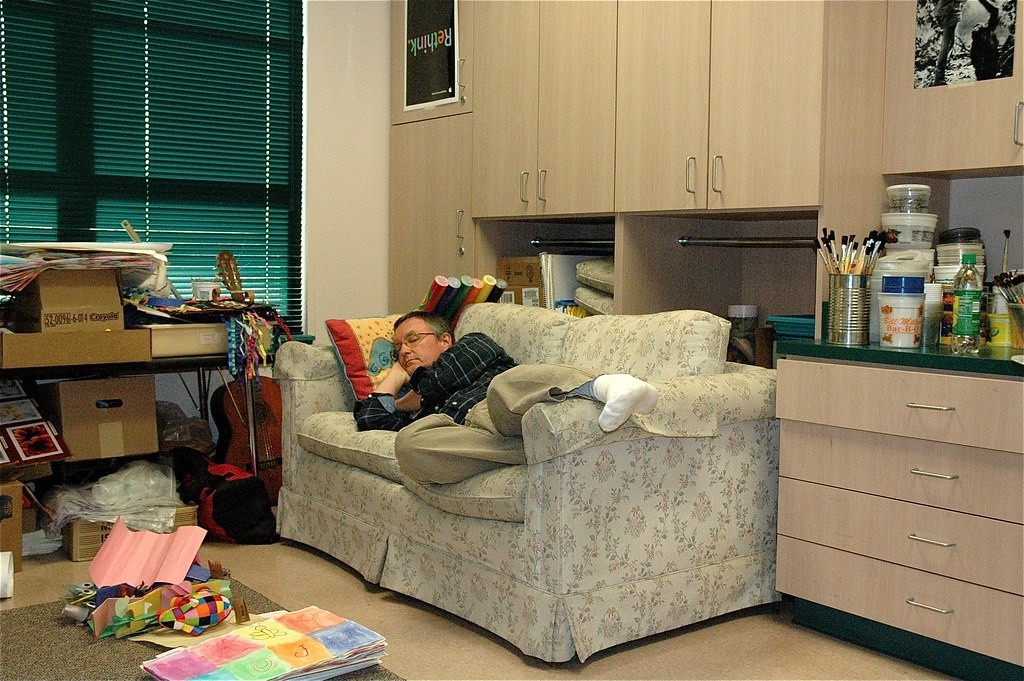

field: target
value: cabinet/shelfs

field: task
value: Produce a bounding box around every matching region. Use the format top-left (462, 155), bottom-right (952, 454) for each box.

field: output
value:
top-left (775, 355), bottom-right (1024, 681)
top-left (390, 0), bottom-right (951, 317)
top-left (882, 1), bottom-right (1024, 175)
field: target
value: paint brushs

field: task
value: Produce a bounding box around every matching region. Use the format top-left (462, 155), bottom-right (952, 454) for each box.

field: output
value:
top-left (813, 227), bottom-right (890, 274)
top-left (993, 268), bottom-right (1024, 305)
top-left (1002, 229), bottom-right (1011, 272)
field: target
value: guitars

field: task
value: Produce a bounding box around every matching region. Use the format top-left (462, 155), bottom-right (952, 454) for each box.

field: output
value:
top-left (210, 252), bottom-right (284, 504)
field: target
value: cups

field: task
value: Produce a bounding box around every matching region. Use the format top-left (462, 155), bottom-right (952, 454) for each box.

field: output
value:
top-left (877, 293), bottom-right (925, 347)
top-left (883, 276), bottom-right (925, 292)
top-left (1009, 303), bottom-right (1024, 349)
top-left (826, 273), bottom-right (872, 347)
top-left (726, 304), bottom-right (757, 364)
top-left (987, 313), bottom-right (1012, 346)
top-left (936, 227), bottom-right (987, 290)
top-left (193, 281), bottom-right (221, 301)
top-left (924, 284), bottom-right (943, 347)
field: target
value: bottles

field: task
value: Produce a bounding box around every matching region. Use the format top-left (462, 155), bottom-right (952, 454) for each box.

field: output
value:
top-left (953, 253), bottom-right (983, 355)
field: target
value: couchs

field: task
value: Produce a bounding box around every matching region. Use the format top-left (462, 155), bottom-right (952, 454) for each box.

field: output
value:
top-left (274, 302), bottom-right (781, 663)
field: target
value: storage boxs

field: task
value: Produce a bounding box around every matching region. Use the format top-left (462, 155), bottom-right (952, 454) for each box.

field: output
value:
top-left (1, 269), bottom-right (151, 368)
top-left (0, 482), bottom-right (23, 572)
top-left (37, 375), bottom-right (159, 462)
top-left (130, 322), bottom-right (229, 358)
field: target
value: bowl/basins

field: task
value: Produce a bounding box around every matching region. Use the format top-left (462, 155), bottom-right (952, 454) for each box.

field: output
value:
top-left (887, 184), bottom-right (930, 213)
top-left (881, 213), bottom-right (938, 250)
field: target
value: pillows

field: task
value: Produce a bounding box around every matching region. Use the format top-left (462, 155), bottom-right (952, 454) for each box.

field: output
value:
top-left (574, 258), bottom-right (614, 315)
top-left (326, 313), bottom-right (405, 402)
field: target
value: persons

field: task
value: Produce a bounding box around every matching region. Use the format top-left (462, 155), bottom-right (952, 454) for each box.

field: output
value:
top-left (353, 310), bottom-right (659, 485)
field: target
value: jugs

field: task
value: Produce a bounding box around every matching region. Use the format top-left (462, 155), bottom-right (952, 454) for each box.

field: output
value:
top-left (871, 251), bottom-right (931, 342)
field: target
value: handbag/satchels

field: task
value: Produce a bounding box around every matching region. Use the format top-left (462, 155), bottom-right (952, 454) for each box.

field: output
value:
top-left (180, 463), bottom-right (279, 546)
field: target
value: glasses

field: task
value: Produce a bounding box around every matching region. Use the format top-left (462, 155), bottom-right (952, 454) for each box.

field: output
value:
top-left (391, 332), bottom-right (435, 354)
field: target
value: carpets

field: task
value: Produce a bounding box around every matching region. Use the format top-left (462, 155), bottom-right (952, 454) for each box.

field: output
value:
top-left (1, 576), bottom-right (406, 681)
